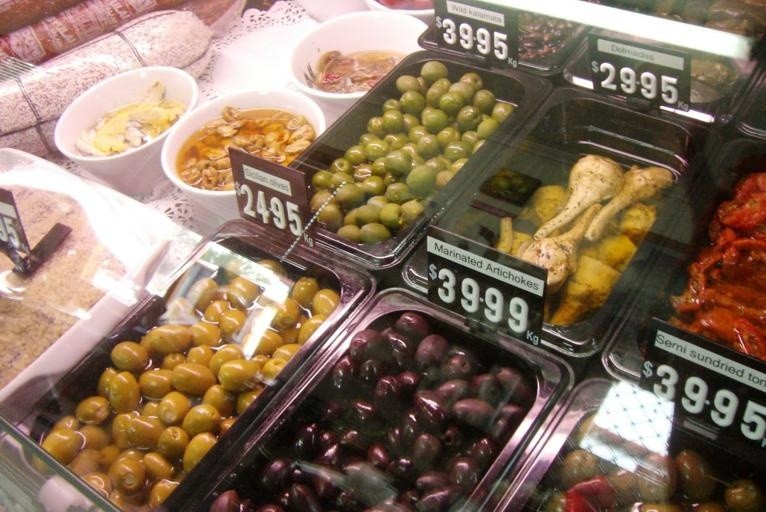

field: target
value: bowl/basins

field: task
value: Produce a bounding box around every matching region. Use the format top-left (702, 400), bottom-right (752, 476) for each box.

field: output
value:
top-left (286, 9), bottom-right (432, 123)
top-left (157, 86), bottom-right (328, 221)
top-left (54, 66), bottom-right (198, 198)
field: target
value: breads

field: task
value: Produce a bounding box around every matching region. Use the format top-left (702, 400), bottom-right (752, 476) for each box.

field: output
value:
top-left (1, 0), bottom-right (247, 156)
top-left (1, 185), bottom-right (125, 388)
